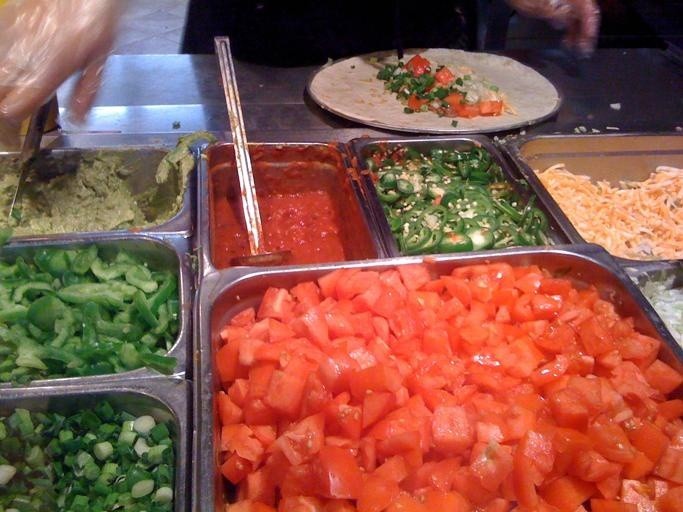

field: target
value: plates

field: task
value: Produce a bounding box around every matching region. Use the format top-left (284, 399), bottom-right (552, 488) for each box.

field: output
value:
top-left (306, 47), bottom-right (565, 136)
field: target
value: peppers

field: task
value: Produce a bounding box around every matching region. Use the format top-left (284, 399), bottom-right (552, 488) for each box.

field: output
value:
top-left (362, 143), bottom-right (551, 255)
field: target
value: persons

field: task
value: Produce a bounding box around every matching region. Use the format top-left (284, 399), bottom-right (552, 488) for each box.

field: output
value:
top-left (0, 0), bottom-right (599, 125)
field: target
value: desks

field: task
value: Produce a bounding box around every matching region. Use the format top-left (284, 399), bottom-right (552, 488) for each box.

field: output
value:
top-left (54, 48), bottom-right (683, 134)
